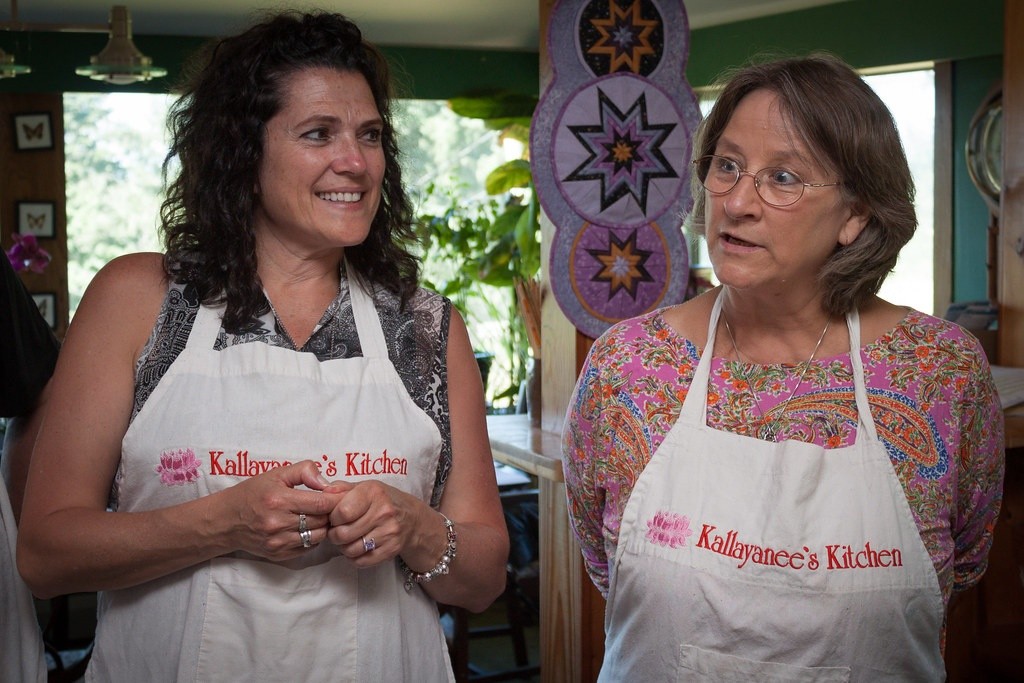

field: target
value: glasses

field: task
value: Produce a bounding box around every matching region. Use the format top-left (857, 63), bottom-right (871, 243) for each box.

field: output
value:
top-left (691, 155), bottom-right (842, 206)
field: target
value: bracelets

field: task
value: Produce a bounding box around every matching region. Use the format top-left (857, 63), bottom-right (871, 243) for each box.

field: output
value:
top-left (399, 512), bottom-right (458, 593)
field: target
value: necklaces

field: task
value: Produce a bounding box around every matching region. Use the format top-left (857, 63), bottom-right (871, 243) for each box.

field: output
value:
top-left (720, 309), bottom-right (834, 447)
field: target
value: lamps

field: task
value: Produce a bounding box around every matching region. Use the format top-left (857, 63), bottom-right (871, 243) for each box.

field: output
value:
top-left (0, 0), bottom-right (167, 86)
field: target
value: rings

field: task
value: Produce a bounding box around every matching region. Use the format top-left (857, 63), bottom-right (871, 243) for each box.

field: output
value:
top-left (298, 514), bottom-right (307, 533)
top-left (299, 530), bottom-right (312, 547)
top-left (362, 536), bottom-right (376, 553)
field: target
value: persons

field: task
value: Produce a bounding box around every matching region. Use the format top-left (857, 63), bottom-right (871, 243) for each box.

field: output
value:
top-left (562, 47), bottom-right (1007, 683)
top-left (0, 249), bottom-right (62, 526)
top-left (16, 13), bottom-right (509, 683)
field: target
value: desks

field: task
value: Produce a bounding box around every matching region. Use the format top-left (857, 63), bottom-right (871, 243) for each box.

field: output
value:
top-left (438, 411), bottom-right (541, 683)
